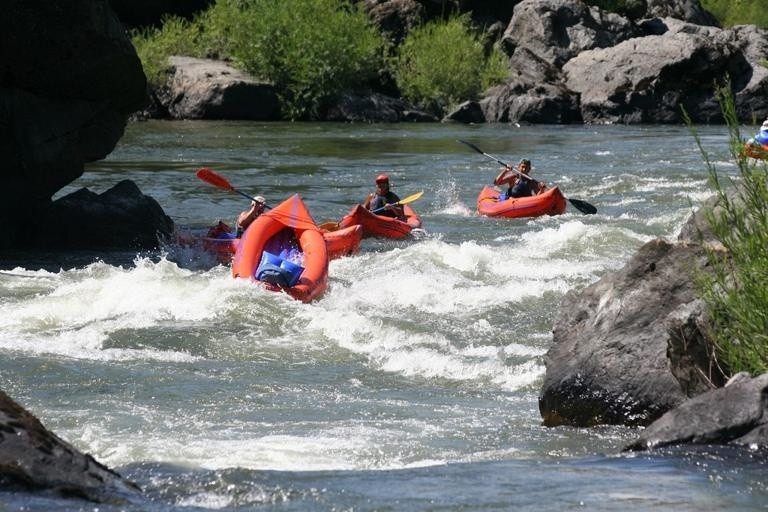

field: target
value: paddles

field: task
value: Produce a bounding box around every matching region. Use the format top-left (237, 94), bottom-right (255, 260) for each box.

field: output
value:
top-left (196, 167), bottom-right (274, 210)
top-left (316, 188), bottom-right (425, 229)
top-left (458, 138), bottom-right (597, 214)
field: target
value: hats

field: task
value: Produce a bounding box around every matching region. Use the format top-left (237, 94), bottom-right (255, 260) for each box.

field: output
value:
top-left (251, 196), bottom-right (266, 206)
top-left (759, 120), bottom-right (768, 132)
top-left (376, 175), bottom-right (388, 183)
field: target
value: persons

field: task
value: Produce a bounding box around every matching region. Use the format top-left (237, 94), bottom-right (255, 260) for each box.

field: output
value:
top-left (364, 174), bottom-right (404, 218)
top-left (493, 158), bottom-right (546, 200)
top-left (235, 195), bottom-right (267, 236)
top-left (757, 119), bottom-right (768, 144)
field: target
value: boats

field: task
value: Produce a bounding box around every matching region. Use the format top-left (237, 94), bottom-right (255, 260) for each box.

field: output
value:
top-left (472, 180), bottom-right (566, 220)
top-left (230, 191), bottom-right (327, 305)
top-left (179, 225), bottom-right (364, 267)
top-left (336, 205), bottom-right (422, 240)
top-left (736, 140), bottom-right (768, 160)
